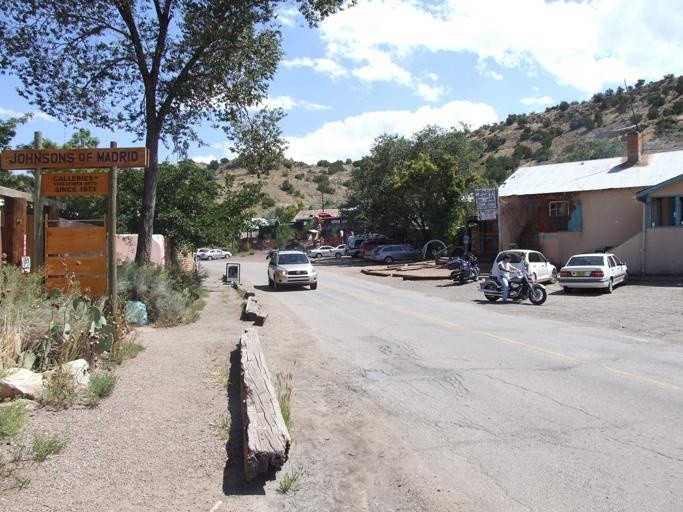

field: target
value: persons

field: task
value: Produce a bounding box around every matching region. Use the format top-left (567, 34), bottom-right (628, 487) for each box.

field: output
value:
top-left (519, 252), bottom-right (528, 274)
top-left (496, 254), bottom-right (520, 302)
top-left (469, 255), bottom-right (480, 281)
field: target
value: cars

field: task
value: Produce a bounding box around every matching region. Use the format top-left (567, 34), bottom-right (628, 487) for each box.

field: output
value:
top-left (307, 233), bottom-right (420, 264)
top-left (491, 248), bottom-right (632, 294)
top-left (193, 247), bottom-right (232, 261)
top-left (268, 250), bottom-right (318, 291)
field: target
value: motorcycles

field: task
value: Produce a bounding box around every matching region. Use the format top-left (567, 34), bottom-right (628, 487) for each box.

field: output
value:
top-left (450, 255), bottom-right (547, 305)
